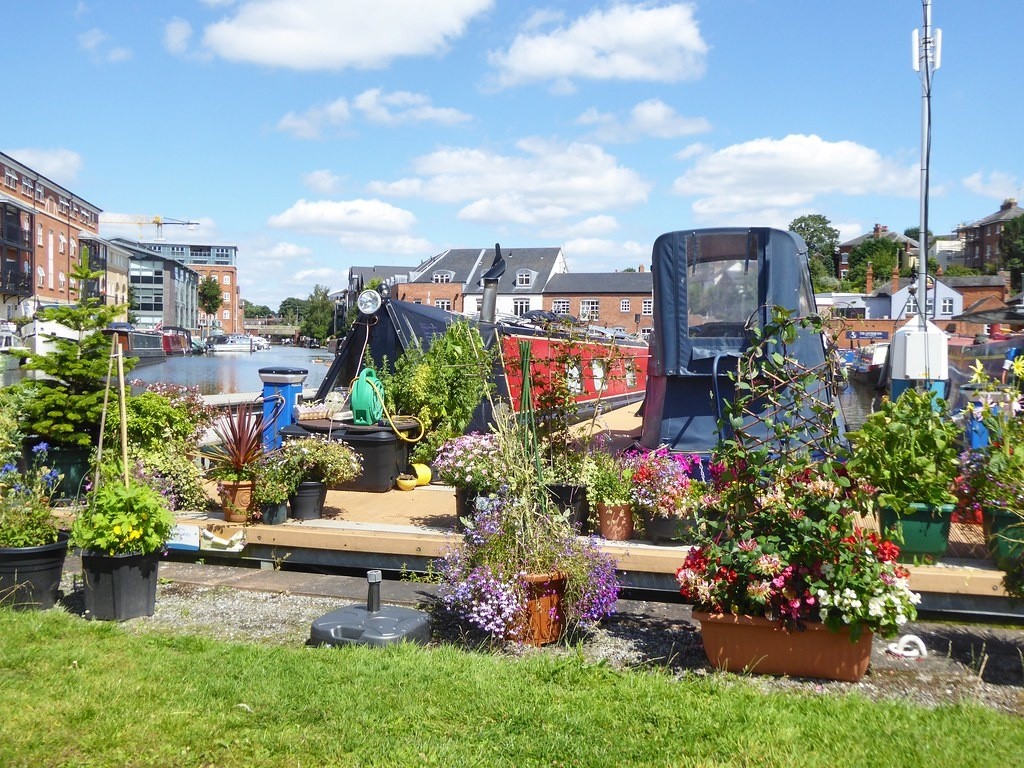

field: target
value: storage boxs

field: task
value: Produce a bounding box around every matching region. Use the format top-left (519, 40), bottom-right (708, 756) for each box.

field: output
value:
top-left (278, 422), bottom-right (421, 493)
top-left (958, 397), bottom-right (1008, 461)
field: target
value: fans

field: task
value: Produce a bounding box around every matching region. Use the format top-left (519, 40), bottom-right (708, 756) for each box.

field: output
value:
top-left (351, 368), bottom-right (385, 425)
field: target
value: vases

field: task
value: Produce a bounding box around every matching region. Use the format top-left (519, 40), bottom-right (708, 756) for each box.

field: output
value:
top-left (982, 507), bottom-right (1024, 571)
top-left (507, 572), bottom-right (566, 645)
top-left (288, 482), bottom-right (327, 520)
top-left (705, 511), bottom-right (730, 540)
top-left (640, 509), bottom-right (699, 545)
top-left (455, 488), bottom-right (487, 534)
top-left (691, 610), bottom-right (873, 683)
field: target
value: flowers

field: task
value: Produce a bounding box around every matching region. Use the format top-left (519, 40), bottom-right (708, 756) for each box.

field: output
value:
top-left (430, 356), bottom-right (1024, 654)
top-left (84, 374), bottom-right (365, 519)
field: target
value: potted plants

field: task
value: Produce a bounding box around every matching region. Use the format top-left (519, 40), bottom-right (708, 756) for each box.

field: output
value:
top-left (185, 400), bottom-right (281, 522)
top-left (842, 386), bottom-right (968, 556)
top-left (251, 466), bottom-right (287, 525)
top-left (522, 306), bottom-right (642, 535)
top-left (0, 447), bottom-right (73, 613)
top-left (586, 451), bottom-right (636, 541)
top-left (68, 480), bottom-right (177, 623)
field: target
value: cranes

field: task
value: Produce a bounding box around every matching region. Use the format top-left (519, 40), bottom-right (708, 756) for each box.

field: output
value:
top-left (99, 213), bottom-right (200, 244)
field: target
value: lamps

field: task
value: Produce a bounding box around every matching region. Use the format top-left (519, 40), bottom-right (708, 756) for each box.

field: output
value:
top-left (357, 284), bottom-right (392, 319)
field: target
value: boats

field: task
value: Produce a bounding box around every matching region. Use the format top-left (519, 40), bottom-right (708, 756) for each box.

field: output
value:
top-left (21, 297), bottom-right (204, 391)
